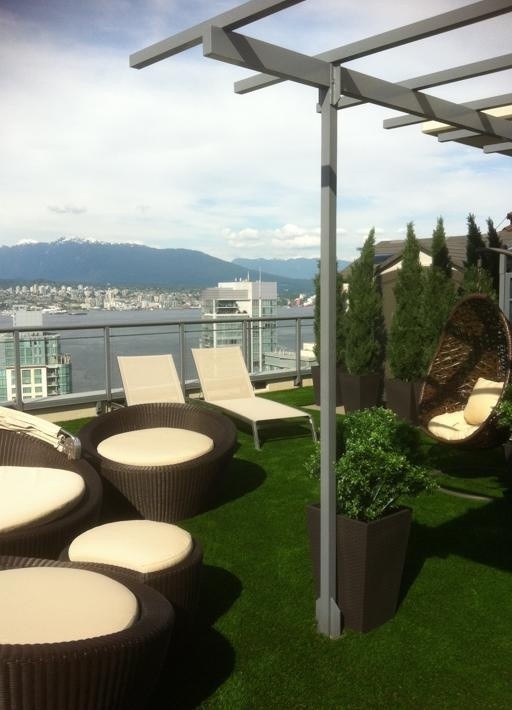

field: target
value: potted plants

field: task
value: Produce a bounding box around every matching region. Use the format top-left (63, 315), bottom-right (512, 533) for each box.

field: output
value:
top-left (304, 403), bottom-right (438, 637)
top-left (313, 217), bottom-right (455, 429)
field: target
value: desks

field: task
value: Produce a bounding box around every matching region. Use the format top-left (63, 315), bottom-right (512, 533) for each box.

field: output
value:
top-left (55, 516), bottom-right (206, 624)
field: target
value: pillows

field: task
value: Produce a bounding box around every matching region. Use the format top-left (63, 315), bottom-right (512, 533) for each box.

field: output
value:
top-left (462, 376), bottom-right (503, 427)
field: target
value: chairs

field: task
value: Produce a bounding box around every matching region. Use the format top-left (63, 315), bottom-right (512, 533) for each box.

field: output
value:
top-left (1, 551), bottom-right (180, 710)
top-left (1, 402), bottom-right (104, 558)
top-left (76, 400), bottom-right (238, 523)
top-left (112, 344), bottom-right (319, 451)
